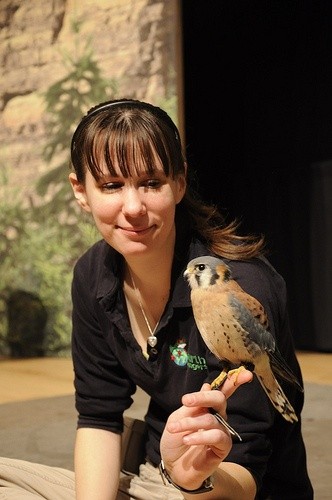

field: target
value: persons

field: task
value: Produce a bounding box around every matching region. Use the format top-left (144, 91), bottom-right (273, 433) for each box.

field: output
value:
top-left (0, 97), bottom-right (316, 500)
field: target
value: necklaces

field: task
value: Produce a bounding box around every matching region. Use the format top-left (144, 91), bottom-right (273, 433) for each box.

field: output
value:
top-left (127, 265), bottom-right (169, 347)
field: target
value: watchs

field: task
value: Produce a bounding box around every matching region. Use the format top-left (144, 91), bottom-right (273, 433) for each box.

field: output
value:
top-left (157, 457), bottom-right (214, 495)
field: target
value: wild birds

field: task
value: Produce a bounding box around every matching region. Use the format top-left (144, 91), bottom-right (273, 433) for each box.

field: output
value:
top-left (183, 255), bottom-right (305, 443)
